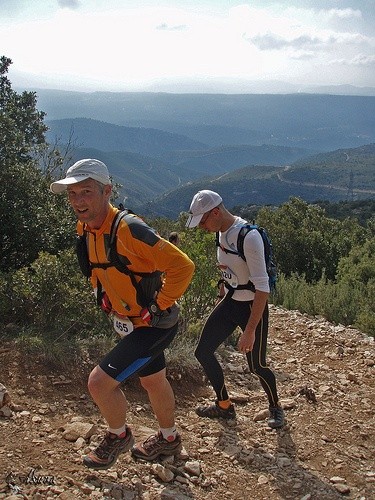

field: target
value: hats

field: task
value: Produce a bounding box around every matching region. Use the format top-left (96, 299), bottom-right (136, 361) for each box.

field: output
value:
top-left (50, 159), bottom-right (111, 194)
top-left (185, 190), bottom-right (222, 228)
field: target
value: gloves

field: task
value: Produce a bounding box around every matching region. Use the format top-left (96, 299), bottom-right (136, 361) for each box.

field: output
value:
top-left (140, 299), bottom-right (163, 328)
top-left (99, 291), bottom-right (112, 315)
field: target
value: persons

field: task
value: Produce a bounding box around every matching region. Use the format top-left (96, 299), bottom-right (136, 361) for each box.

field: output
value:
top-left (50, 158), bottom-right (195, 468)
top-left (184, 190), bottom-right (286, 428)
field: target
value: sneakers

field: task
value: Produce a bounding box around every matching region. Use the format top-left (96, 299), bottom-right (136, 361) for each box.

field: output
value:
top-left (83, 424), bottom-right (135, 469)
top-left (268, 402), bottom-right (284, 427)
top-left (195, 400), bottom-right (236, 419)
top-left (130, 430), bottom-right (182, 461)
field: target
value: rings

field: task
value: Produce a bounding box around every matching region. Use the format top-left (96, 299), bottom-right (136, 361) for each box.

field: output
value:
top-left (246, 348), bottom-right (250, 351)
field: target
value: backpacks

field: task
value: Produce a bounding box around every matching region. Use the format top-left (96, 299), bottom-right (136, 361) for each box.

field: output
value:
top-left (216, 223), bottom-right (276, 294)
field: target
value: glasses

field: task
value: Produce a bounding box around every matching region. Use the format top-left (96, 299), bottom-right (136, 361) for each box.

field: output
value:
top-left (197, 207), bottom-right (219, 229)
